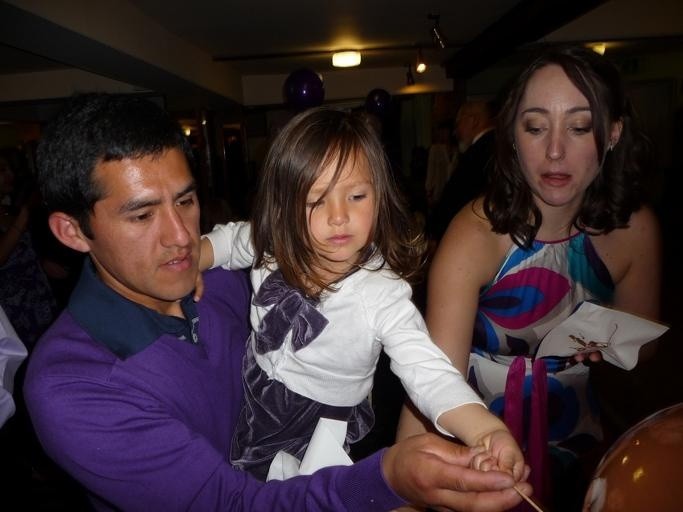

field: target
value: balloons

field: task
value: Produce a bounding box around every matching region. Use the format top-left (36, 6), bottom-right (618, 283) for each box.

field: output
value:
top-left (365, 89), bottom-right (390, 116)
top-left (284, 67), bottom-right (324, 114)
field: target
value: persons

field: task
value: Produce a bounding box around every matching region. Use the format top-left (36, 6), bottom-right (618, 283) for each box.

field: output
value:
top-left (392, 44), bottom-right (665, 512)
top-left (420, 94), bottom-right (514, 264)
top-left (409, 118), bottom-right (461, 226)
top-left (19, 92), bottom-right (535, 511)
top-left (191, 104), bottom-right (528, 486)
top-left (0, 144), bottom-right (66, 353)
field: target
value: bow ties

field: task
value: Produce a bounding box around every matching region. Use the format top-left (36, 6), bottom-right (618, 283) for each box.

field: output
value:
top-left (252, 268), bottom-right (330, 356)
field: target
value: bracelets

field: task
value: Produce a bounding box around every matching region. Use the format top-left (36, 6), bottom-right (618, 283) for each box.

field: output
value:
top-left (11, 222), bottom-right (25, 236)
